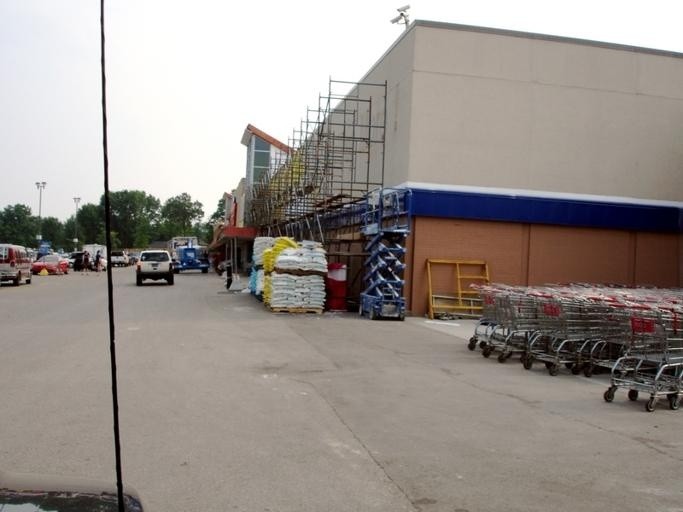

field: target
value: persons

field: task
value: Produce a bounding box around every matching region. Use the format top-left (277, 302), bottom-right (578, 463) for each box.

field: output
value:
top-left (94, 250), bottom-right (101, 272)
top-left (81, 251), bottom-right (89, 273)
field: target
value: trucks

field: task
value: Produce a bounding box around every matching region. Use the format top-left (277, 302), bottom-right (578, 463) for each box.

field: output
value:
top-left (81, 242), bottom-right (107, 271)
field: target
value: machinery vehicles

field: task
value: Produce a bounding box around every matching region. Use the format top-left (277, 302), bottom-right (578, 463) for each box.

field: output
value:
top-left (169, 237), bottom-right (210, 273)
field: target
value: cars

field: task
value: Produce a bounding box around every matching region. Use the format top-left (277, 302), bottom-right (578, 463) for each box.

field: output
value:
top-left (128, 251), bottom-right (141, 267)
top-left (64, 252), bottom-right (86, 268)
top-left (32, 254), bottom-right (70, 274)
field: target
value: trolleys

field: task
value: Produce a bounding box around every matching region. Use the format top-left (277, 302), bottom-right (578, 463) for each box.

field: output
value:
top-left (469, 281), bottom-right (683, 412)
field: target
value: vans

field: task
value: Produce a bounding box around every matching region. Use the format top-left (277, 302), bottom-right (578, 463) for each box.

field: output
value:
top-left (0, 243), bottom-right (32, 286)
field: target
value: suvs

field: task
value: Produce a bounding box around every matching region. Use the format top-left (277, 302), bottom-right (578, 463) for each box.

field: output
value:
top-left (111, 251), bottom-right (129, 267)
top-left (136, 250), bottom-right (175, 286)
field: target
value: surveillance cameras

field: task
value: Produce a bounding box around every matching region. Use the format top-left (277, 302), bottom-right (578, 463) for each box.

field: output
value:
top-left (397, 4), bottom-right (410, 12)
top-left (391, 13), bottom-right (404, 24)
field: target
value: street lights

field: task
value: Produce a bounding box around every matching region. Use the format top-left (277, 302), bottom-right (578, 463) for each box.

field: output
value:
top-left (72, 197), bottom-right (81, 251)
top-left (35, 181), bottom-right (46, 246)
